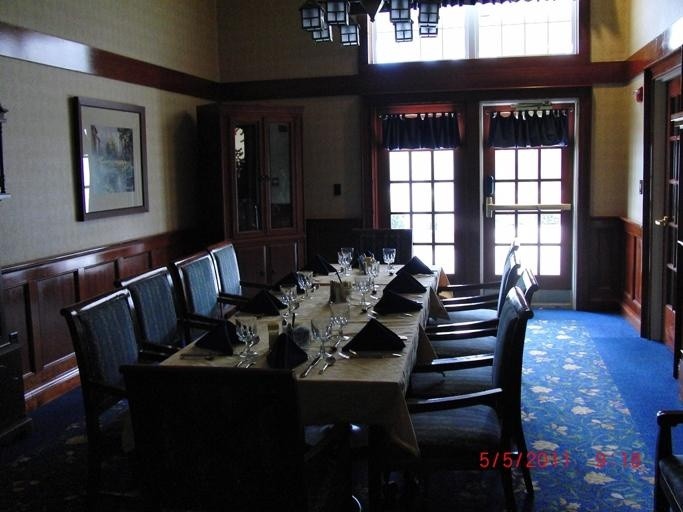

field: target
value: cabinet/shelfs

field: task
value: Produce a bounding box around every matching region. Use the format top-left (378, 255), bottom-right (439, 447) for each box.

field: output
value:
top-left (197, 104), bottom-right (306, 292)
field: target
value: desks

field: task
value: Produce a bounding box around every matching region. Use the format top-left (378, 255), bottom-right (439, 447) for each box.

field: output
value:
top-left (159, 264), bottom-right (443, 512)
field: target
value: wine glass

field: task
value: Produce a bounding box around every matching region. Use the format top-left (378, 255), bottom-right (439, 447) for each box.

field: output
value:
top-left (296, 270), bottom-right (314, 302)
top-left (338, 248), bottom-right (353, 277)
top-left (308, 316), bottom-right (336, 357)
top-left (355, 260), bottom-right (380, 310)
top-left (328, 301), bottom-right (351, 343)
top-left (382, 246), bottom-right (396, 272)
top-left (280, 283), bottom-right (298, 318)
top-left (233, 315), bottom-right (258, 358)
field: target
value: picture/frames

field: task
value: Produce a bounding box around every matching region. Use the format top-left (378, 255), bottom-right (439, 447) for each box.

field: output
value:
top-left (74, 95), bottom-right (148, 222)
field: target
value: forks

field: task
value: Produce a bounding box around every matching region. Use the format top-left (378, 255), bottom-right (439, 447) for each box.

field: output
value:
top-left (367, 311), bottom-right (414, 320)
top-left (337, 350), bottom-right (404, 361)
top-left (329, 339), bottom-right (340, 351)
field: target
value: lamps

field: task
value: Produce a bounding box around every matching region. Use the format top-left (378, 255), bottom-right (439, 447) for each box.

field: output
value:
top-left (298, 0), bottom-right (440, 48)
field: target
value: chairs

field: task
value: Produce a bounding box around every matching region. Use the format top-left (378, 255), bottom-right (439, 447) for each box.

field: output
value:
top-left (368, 240), bottom-right (540, 512)
top-left (655, 410), bottom-right (683, 512)
top-left (209, 244), bottom-right (276, 315)
top-left (120, 266), bottom-right (217, 357)
top-left (60, 289), bottom-right (171, 512)
top-left (172, 251), bottom-right (224, 341)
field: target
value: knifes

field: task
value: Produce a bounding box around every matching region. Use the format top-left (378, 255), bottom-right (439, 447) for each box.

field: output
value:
top-left (299, 357), bottom-right (321, 378)
top-left (178, 352), bottom-right (227, 358)
top-left (234, 359), bottom-right (256, 369)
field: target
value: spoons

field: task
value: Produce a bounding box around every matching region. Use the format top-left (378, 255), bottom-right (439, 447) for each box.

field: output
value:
top-left (320, 356), bottom-right (336, 376)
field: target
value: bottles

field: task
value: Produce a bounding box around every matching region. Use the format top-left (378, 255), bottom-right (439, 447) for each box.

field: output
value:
top-left (281, 318), bottom-right (288, 335)
top-left (267, 322), bottom-right (279, 351)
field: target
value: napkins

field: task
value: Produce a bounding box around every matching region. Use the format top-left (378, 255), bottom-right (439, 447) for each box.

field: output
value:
top-left (396, 256), bottom-right (433, 278)
top-left (374, 287), bottom-right (423, 314)
top-left (344, 317), bottom-right (405, 353)
top-left (196, 321), bottom-right (253, 355)
top-left (252, 290), bottom-right (289, 319)
top-left (381, 269), bottom-right (425, 296)
top-left (278, 271), bottom-right (311, 294)
top-left (303, 250), bottom-right (336, 277)
